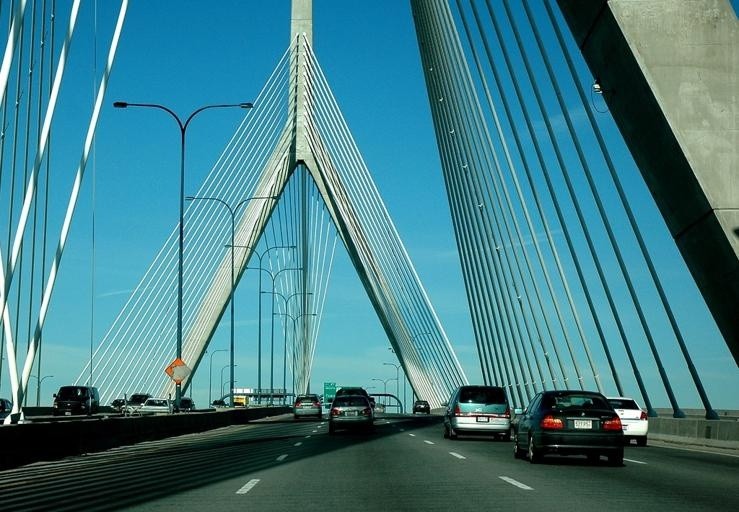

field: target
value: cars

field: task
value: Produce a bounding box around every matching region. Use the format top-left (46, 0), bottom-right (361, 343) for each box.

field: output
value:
top-left (514, 391), bottom-right (624, 467)
top-left (328, 387), bottom-right (375, 435)
top-left (293, 396), bottom-right (323, 419)
top-left (0, 398), bottom-right (13, 425)
top-left (110, 393), bottom-right (195, 415)
top-left (606, 396), bottom-right (648, 446)
top-left (413, 401), bottom-right (430, 414)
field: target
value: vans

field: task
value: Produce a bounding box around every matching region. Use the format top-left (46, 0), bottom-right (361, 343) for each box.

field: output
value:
top-left (442, 385), bottom-right (511, 442)
top-left (53, 385), bottom-right (99, 416)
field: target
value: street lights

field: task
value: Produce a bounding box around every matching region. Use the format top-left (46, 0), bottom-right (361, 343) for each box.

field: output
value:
top-left (372, 378), bottom-right (397, 412)
top-left (114, 100), bottom-right (253, 414)
top-left (30, 374), bottom-right (54, 406)
top-left (185, 196), bottom-right (317, 408)
top-left (384, 363), bottom-right (402, 413)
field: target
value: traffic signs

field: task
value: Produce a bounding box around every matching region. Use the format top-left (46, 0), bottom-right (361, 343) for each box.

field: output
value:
top-left (324, 383), bottom-right (336, 403)
